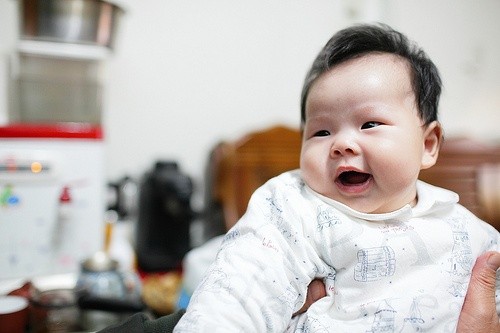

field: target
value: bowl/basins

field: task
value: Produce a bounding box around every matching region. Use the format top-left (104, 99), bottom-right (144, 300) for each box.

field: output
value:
top-left (18, 0), bottom-right (126, 50)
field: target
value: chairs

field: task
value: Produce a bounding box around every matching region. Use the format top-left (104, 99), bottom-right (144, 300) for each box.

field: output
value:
top-left (214, 125), bottom-right (310, 233)
top-left (411, 136), bottom-right (500, 231)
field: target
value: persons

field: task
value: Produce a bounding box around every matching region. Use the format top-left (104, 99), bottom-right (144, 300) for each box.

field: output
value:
top-left (173, 23), bottom-right (500, 333)
top-left (293, 251), bottom-right (498, 333)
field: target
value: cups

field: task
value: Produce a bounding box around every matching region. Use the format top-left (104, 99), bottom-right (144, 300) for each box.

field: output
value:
top-left (0, 295), bottom-right (29, 333)
top-left (26, 288), bottom-right (89, 333)
top-left (73, 252), bottom-right (142, 310)
